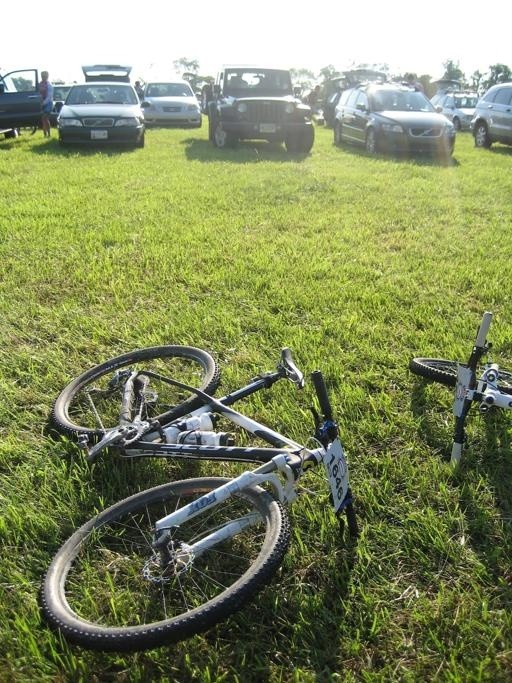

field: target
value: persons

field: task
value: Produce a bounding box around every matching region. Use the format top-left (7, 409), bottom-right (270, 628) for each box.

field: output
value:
top-left (39, 70), bottom-right (54, 137)
top-left (134, 80), bottom-right (145, 94)
top-left (301, 84), bottom-right (321, 118)
top-left (407, 72), bottom-right (425, 94)
